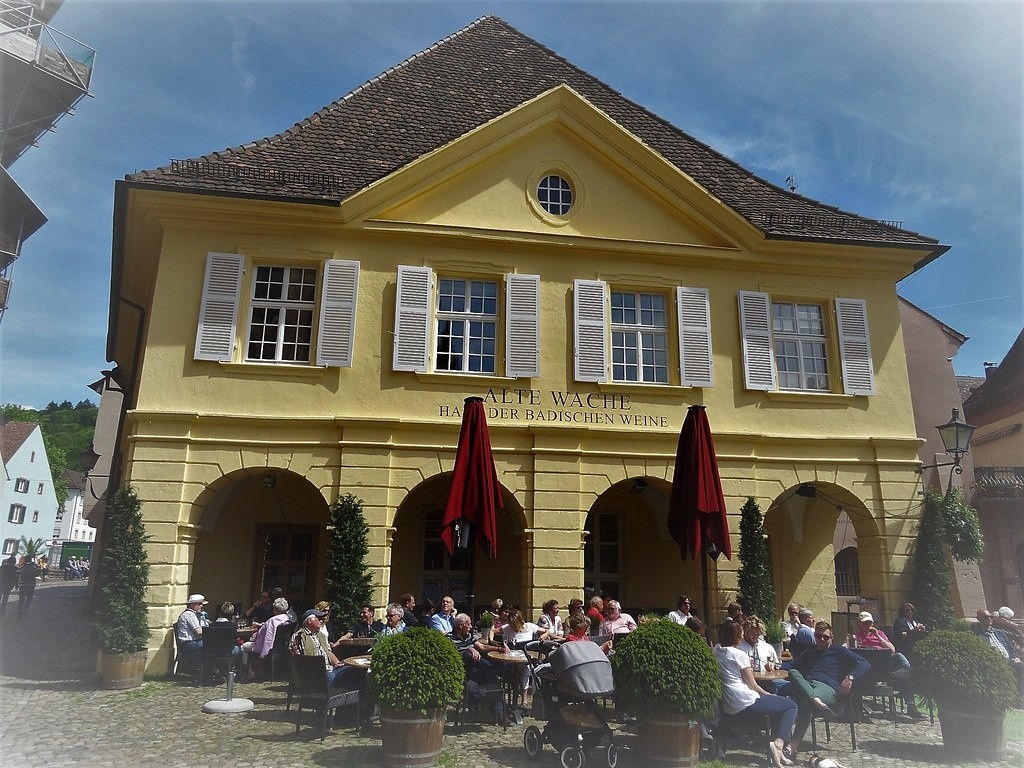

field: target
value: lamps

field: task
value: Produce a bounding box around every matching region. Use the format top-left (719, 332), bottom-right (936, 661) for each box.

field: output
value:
top-left (81, 444), bottom-right (109, 501)
top-left (916, 408), bottom-right (977, 495)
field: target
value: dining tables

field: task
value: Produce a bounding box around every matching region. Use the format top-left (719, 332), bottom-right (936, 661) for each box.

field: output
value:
top-left (752, 668), bottom-right (788, 747)
top-left (486, 649), bottom-right (545, 726)
top-left (344, 654), bottom-right (372, 719)
top-left (237, 627), bottom-right (259, 641)
top-left (338, 636), bottom-right (376, 645)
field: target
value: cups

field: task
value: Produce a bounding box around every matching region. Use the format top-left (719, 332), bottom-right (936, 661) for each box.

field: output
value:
top-left (347, 625), bottom-right (353, 633)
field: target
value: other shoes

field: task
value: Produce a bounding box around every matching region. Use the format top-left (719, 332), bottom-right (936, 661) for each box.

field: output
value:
top-left (234, 677), bottom-right (248, 683)
top-left (616, 713), bottom-right (636, 725)
top-left (820, 706), bottom-right (837, 722)
top-left (699, 723), bottom-right (713, 742)
top-left (498, 717), bottom-right (516, 727)
top-left (768, 742), bottom-right (793, 768)
top-left (521, 702), bottom-right (529, 709)
top-left (907, 704), bottom-right (929, 720)
top-left (783, 748), bottom-right (798, 764)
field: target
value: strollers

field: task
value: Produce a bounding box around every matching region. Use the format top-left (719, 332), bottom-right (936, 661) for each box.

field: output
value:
top-left (524, 637), bottom-right (619, 768)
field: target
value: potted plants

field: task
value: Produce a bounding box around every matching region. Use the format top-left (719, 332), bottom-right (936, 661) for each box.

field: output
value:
top-left (613, 618), bottom-right (722, 768)
top-left (92, 483), bottom-right (157, 687)
top-left (911, 631), bottom-right (1020, 759)
top-left (371, 627), bottom-right (466, 768)
top-left (478, 612), bottom-right (494, 639)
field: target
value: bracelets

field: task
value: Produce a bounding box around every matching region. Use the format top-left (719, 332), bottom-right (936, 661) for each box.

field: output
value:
top-left (846, 674), bottom-right (855, 680)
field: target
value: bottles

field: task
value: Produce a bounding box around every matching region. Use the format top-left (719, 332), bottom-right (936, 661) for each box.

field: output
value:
top-left (754, 649), bottom-right (760, 671)
top-left (850, 623), bottom-right (856, 649)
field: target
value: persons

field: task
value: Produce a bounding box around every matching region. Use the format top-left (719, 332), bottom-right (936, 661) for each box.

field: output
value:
top-left (779, 603), bottom-right (817, 645)
top-left (0, 555), bottom-right (49, 618)
top-left (444, 613), bottom-right (512, 727)
top-left (352, 605), bottom-right (384, 638)
top-left (711, 602), bottom-right (798, 768)
top-left (401, 593), bottom-right (457, 637)
top-left (894, 603), bottom-right (927, 641)
top-left (841, 611), bottom-right (922, 718)
top-left (176, 593), bottom-right (213, 653)
top-left (288, 601), bottom-right (367, 691)
top-left (209, 600), bottom-right (241, 685)
top-left (369, 603), bottom-right (410, 651)
top-left (667, 594), bottom-right (707, 637)
top-left (489, 598), bottom-right (552, 709)
top-left (764, 621), bottom-right (871, 760)
top-left (242, 591), bottom-right (273, 623)
top-left (241, 597), bottom-right (294, 680)
top-left (537, 594), bottom-right (639, 656)
top-left (65, 556), bottom-right (90, 581)
top-left (976, 605), bottom-right (1024, 678)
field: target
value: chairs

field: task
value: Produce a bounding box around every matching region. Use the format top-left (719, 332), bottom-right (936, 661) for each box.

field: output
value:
top-left (174, 603), bottom-right (936, 752)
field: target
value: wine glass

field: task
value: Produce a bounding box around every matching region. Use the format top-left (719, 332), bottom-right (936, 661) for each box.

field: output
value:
top-left (238, 618), bottom-right (255, 630)
top-left (768, 652), bottom-right (782, 674)
top-left (503, 634), bottom-right (516, 655)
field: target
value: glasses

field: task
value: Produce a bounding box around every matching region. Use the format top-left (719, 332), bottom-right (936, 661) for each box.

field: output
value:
top-left (322, 609), bottom-right (330, 614)
top-left (815, 633), bottom-right (833, 640)
top-left (385, 614), bottom-right (398, 618)
top-left (903, 608), bottom-right (914, 613)
top-left (194, 603), bottom-right (203, 606)
top-left (607, 608), bottom-right (618, 611)
top-left (981, 615), bottom-right (993, 620)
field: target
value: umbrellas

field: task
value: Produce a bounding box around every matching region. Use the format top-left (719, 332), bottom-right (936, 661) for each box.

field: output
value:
top-left (667, 405), bottom-right (731, 637)
top-left (439, 396), bottom-right (504, 634)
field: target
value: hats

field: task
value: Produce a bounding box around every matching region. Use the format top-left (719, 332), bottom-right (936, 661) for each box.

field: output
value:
top-left (72, 555), bottom-right (76, 559)
top-left (184, 594), bottom-right (209, 605)
top-left (858, 611), bottom-right (874, 623)
top-left (302, 609), bottom-right (326, 622)
